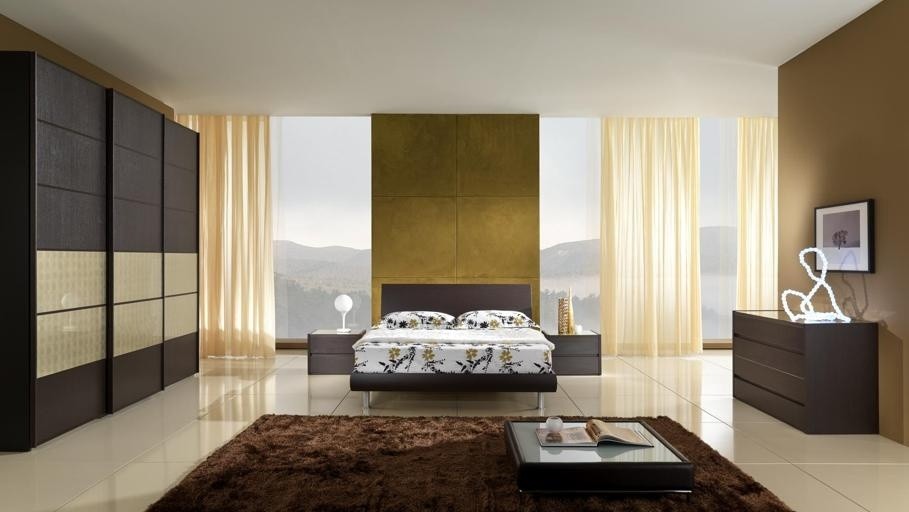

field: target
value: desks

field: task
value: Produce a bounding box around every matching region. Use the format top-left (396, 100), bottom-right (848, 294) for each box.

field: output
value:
top-left (730, 304), bottom-right (882, 437)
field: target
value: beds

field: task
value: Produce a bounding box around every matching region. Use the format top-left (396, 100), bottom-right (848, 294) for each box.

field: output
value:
top-left (349, 282), bottom-right (559, 412)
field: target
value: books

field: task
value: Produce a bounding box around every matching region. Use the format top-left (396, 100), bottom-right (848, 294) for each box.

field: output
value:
top-left (534, 418), bottom-right (655, 447)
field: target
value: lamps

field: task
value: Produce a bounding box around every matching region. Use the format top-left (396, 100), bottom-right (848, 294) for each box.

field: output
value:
top-left (334, 294), bottom-right (353, 333)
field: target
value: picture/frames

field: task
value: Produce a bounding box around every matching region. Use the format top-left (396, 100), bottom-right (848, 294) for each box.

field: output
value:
top-left (813, 200), bottom-right (878, 276)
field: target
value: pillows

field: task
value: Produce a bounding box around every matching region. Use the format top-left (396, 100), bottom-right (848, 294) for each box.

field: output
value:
top-left (454, 310), bottom-right (540, 329)
top-left (382, 308), bottom-right (455, 330)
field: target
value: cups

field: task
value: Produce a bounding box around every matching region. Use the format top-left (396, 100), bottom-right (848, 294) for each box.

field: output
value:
top-left (558, 298), bottom-right (568, 334)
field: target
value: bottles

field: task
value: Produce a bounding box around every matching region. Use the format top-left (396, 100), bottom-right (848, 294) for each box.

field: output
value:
top-left (567, 288), bottom-right (575, 334)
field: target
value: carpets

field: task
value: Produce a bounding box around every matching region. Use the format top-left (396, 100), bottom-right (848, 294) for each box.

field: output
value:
top-left (136, 411), bottom-right (797, 512)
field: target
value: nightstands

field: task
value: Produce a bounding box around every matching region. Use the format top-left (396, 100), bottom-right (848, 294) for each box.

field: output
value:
top-left (539, 324), bottom-right (602, 377)
top-left (306, 327), bottom-right (365, 376)
